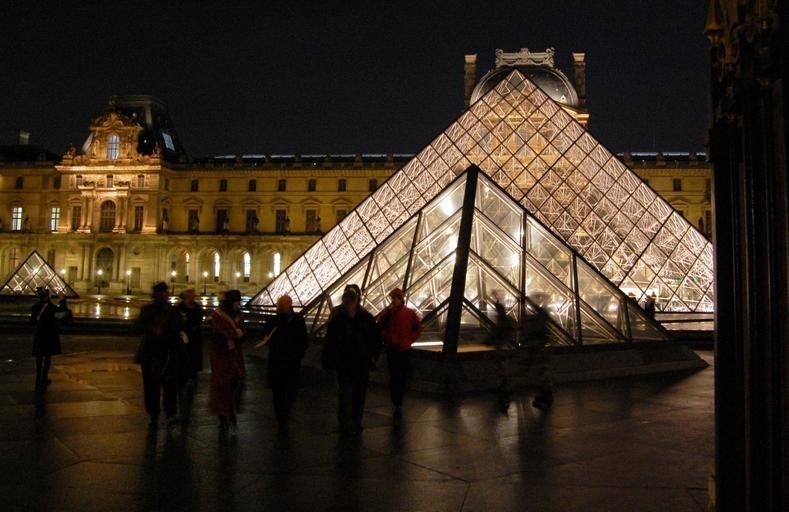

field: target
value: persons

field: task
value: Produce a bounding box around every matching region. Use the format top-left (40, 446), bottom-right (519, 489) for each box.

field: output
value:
top-left (173, 289), bottom-right (206, 425)
top-left (375, 288), bottom-right (423, 426)
top-left (29, 287), bottom-right (69, 386)
top-left (479, 303), bottom-right (554, 417)
top-left (643, 295), bottom-right (656, 319)
top-left (207, 291), bottom-right (248, 446)
top-left (132, 281), bottom-right (184, 445)
top-left (320, 285), bottom-right (381, 451)
top-left (263, 296), bottom-right (308, 450)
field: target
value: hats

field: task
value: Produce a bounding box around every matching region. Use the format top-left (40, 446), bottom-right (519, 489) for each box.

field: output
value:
top-left (179, 288), bottom-right (200, 298)
top-left (152, 282), bottom-right (169, 297)
top-left (37, 286), bottom-right (50, 298)
top-left (389, 288), bottom-right (404, 300)
top-left (342, 284), bottom-right (361, 298)
top-left (221, 290), bottom-right (243, 302)
